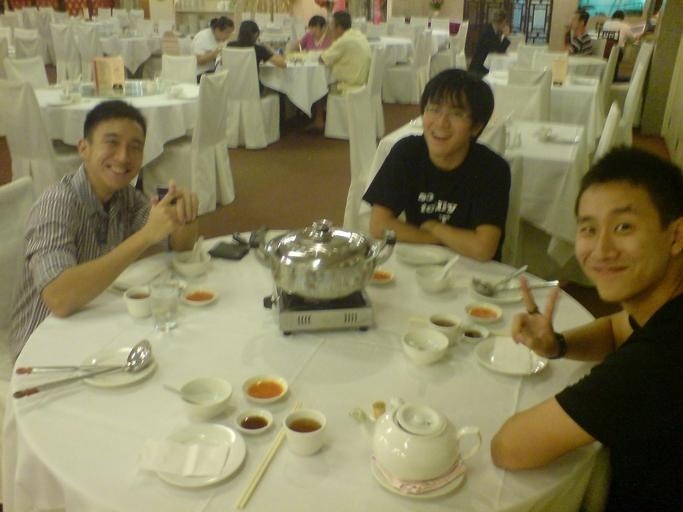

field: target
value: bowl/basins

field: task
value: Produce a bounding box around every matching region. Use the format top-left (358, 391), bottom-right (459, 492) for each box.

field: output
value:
top-left (179, 380), bottom-right (232, 418)
top-left (402, 329), bottom-right (450, 364)
top-left (173, 252), bottom-right (210, 276)
top-left (63, 80), bottom-right (81, 93)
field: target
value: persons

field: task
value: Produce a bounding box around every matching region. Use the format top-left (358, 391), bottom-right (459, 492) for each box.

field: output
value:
top-left (492, 144), bottom-right (683, 512)
top-left (10, 99), bottom-right (199, 362)
top-left (362, 67), bottom-right (509, 263)
top-left (468, 10), bottom-right (509, 76)
top-left (190, 16), bottom-right (233, 84)
top-left (304, 10), bottom-right (372, 132)
top-left (228, 21), bottom-right (286, 119)
top-left (300, 16), bottom-right (330, 52)
top-left (601, 10), bottom-right (636, 62)
top-left (565, 9), bottom-right (591, 56)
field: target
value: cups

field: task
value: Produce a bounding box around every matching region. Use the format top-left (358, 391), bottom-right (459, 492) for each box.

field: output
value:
top-left (124, 286), bottom-right (152, 317)
top-left (429, 314), bottom-right (461, 347)
top-left (551, 55), bottom-right (566, 87)
top-left (149, 280), bottom-right (178, 329)
top-left (285, 410), bottom-right (324, 454)
top-left (71, 94), bottom-right (82, 105)
top-left (154, 72), bottom-right (164, 87)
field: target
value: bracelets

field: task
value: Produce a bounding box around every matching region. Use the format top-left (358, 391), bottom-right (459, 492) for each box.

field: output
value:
top-left (547, 332), bottom-right (567, 359)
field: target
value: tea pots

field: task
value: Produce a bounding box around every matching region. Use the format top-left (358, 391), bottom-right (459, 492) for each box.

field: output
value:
top-left (347, 398), bottom-right (482, 481)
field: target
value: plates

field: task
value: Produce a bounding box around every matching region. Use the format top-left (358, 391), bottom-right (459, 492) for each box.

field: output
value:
top-left (464, 302), bottom-right (501, 322)
top-left (113, 257), bottom-right (168, 290)
top-left (80, 348), bottom-right (156, 387)
top-left (49, 99), bottom-right (72, 108)
top-left (158, 422), bottom-right (246, 487)
top-left (369, 269), bottom-right (393, 285)
top-left (474, 336), bottom-right (548, 376)
top-left (236, 409), bottom-right (272, 434)
top-left (397, 246), bottom-right (447, 264)
top-left (181, 288), bottom-right (217, 305)
top-left (370, 458), bottom-right (465, 500)
top-left (464, 325), bottom-right (488, 341)
top-left (174, 83), bottom-right (200, 101)
top-left (244, 374), bottom-right (289, 402)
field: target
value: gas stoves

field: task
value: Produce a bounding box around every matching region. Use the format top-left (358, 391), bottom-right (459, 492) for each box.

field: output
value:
top-left (262, 282), bottom-right (373, 336)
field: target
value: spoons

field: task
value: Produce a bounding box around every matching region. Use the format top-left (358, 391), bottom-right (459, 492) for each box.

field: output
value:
top-left (13, 340), bottom-right (150, 397)
top-left (417, 265), bottom-right (451, 294)
top-left (474, 264), bottom-right (558, 296)
top-left (164, 385), bottom-right (216, 404)
top-left (427, 255), bottom-right (458, 280)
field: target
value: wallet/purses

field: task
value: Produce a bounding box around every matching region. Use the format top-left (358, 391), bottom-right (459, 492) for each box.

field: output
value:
top-left (207, 242), bottom-right (248, 260)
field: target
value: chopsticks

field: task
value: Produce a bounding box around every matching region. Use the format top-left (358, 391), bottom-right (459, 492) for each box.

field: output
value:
top-left (235, 400), bottom-right (301, 508)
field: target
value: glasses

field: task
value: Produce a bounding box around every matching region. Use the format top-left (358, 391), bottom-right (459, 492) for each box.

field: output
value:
top-left (423, 103), bottom-right (475, 121)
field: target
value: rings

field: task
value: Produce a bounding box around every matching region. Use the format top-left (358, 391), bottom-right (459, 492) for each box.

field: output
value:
top-left (527, 304), bottom-right (537, 315)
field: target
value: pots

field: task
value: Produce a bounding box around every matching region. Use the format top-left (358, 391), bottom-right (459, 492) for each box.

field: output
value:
top-left (251, 219), bottom-right (396, 303)
top-left (257, 37), bottom-right (292, 53)
